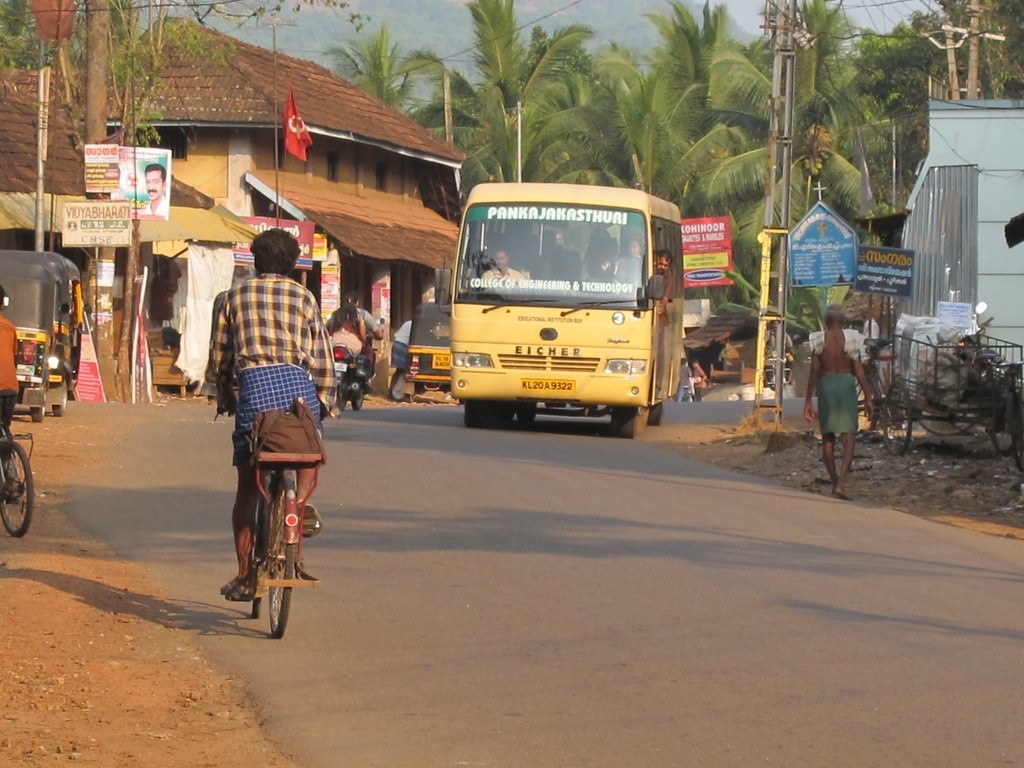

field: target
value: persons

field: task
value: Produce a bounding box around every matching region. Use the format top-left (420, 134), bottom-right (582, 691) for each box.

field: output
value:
top-left (764, 320), bottom-right (794, 387)
top-left (136, 163), bottom-right (168, 216)
top-left (326, 292), bottom-right (386, 382)
top-left (803, 304), bottom-right (874, 499)
top-left (582, 242), bottom-right (621, 283)
top-left (481, 246), bottom-right (527, 288)
top-left (691, 359), bottom-right (706, 401)
top-left (0, 285), bottom-right (24, 505)
top-left (387, 319), bottom-right (413, 399)
top-left (206, 228), bottom-right (340, 602)
top-left (674, 358), bottom-right (692, 401)
top-left (329, 302), bottom-right (366, 355)
top-left (617, 238), bottom-right (643, 285)
top-left (660, 248), bottom-right (674, 314)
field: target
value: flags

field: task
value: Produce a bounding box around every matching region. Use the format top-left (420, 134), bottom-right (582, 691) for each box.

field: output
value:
top-left (284, 85), bottom-right (313, 162)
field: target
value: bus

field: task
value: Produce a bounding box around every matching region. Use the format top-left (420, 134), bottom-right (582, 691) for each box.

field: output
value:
top-left (447, 181), bottom-right (686, 439)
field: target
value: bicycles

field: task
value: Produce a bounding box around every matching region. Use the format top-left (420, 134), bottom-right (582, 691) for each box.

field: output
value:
top-left (853, 337), bottom-right (914, 456)
top-left (0, 389), bottom-right (36, 537)
top-left (204, 386), bottom-right (346, 639)
top-left (682, 385), bottom-right (695, 403)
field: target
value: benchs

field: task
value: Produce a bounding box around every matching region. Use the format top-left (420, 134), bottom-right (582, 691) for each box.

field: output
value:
top-left (151, 357), bottom-right (193, 396)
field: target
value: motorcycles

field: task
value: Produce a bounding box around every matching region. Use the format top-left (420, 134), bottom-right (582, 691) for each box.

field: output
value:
top-left (942, 331), bottom-right (1023, 394)
top-left (760, 345), bottom-right (792, 393)
top-left (333, 317), bottom-right (385, 411)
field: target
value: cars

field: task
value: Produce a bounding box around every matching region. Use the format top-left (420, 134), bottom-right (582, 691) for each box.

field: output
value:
top-left (0, 248), bottom-right (93, 423)
top-left (405, 304), bottom-right (452, 384)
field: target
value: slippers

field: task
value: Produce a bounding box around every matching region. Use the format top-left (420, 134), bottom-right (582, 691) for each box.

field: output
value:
top-left (297, 504), bottom-right (323, 538)
top-left (220, 581), bottom-right (255, 601)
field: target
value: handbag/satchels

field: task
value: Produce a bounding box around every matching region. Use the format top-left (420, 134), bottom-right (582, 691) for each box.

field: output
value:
top-left (253, 398), bottom-right (327, 463)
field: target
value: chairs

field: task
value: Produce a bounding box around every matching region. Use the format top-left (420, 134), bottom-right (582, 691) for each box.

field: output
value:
top-left (542, 249), bottom-right (581, 280)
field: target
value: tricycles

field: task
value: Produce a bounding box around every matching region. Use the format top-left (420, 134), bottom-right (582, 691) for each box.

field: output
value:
top-left (878, 325), bottom-right (1024, 479)
